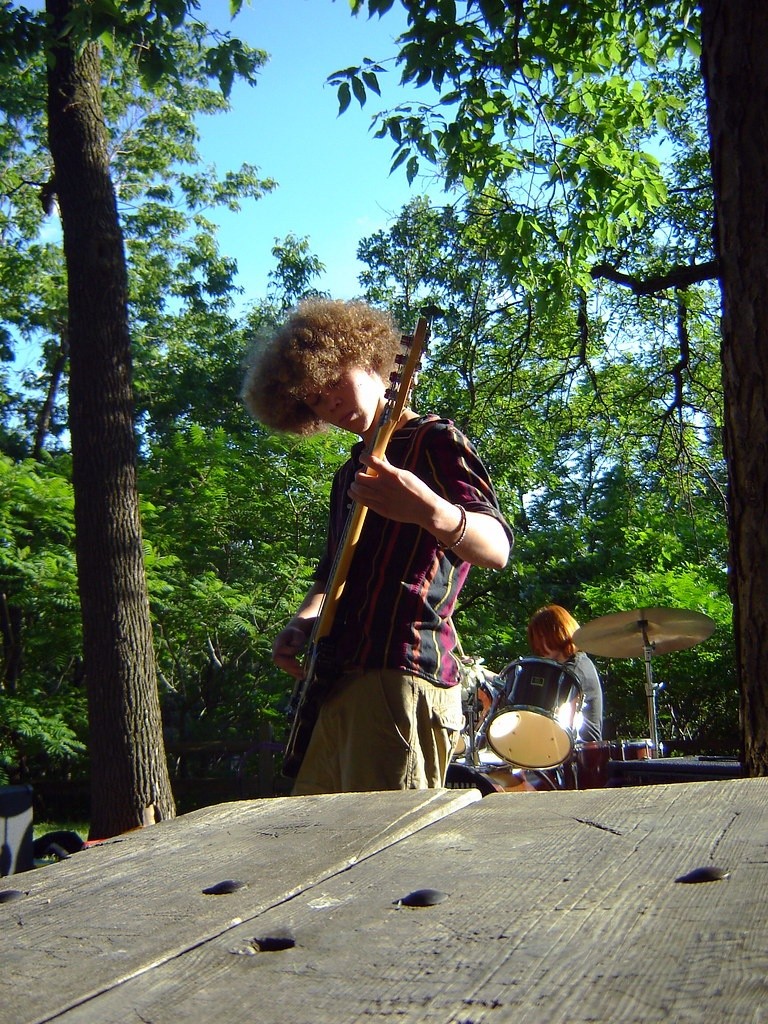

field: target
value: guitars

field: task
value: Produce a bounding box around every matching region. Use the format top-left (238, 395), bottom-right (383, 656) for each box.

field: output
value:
top-left (280, 314), bottom-right (431, 779)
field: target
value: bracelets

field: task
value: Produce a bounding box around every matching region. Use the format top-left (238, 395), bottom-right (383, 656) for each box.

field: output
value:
top-left (436, 504), bottom-right (468, 550)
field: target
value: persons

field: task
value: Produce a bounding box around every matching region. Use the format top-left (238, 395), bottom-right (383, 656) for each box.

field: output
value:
top-left (241, 299), bottom-right (515, 797)
top-left (462, 604), bottom-right (602, 791)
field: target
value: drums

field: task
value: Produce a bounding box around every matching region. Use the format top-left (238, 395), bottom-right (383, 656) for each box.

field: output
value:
top-left (486, 655), bottom-right (584, 772)
top-left (563, 738), bottom-right (653, 790)
top-left (445, 746), bottom-right (558, 798)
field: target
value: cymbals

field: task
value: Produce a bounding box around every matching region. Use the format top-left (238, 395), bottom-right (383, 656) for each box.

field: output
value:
top-left (571, 605), bottom-right (717, 659)
